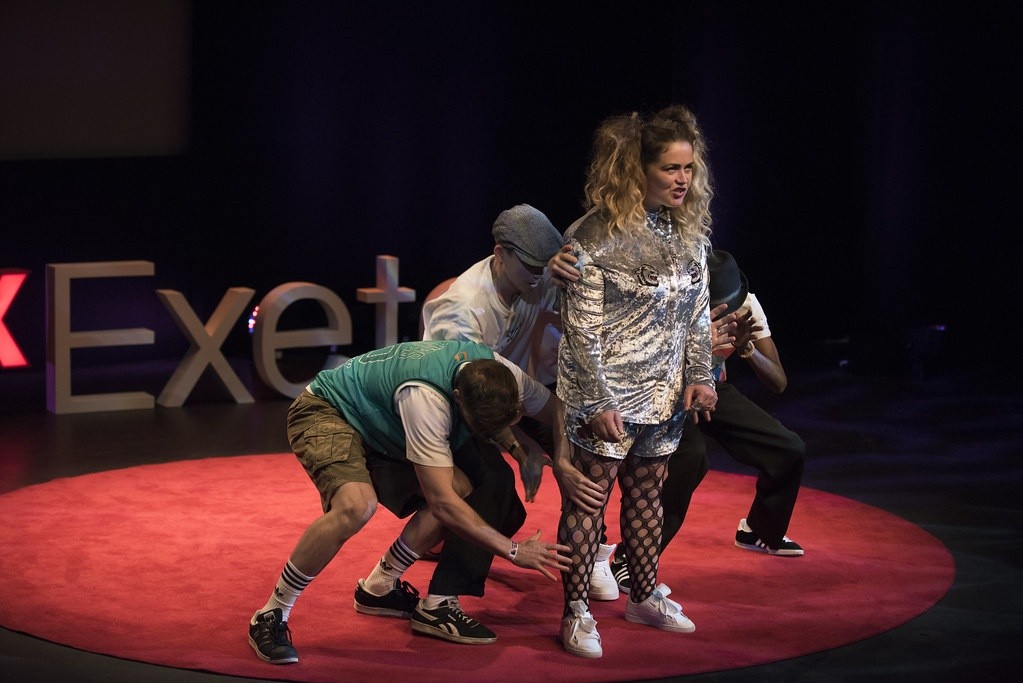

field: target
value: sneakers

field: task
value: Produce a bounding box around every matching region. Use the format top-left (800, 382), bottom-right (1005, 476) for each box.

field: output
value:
top-left (560, 600), bottom-right (603, 657)
top-left (733, 518), bottom-right (805, 556)
top-left (586, 542), bottom-right (619, 601)
top-left (352, 577), bottom-right (418, 617)
top-left (248, 608), bottom-right (300, 664)
top-left (409, 596), bottom-right (498, 644)
top-left (625, 583), bottom-right (695, 633)
top-left (610, 542), bottom-right (636, 593)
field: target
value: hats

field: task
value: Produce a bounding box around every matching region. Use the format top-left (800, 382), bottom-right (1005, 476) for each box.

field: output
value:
top-left (492, 202), bottom-right (565, 267)
top-left (708, 249), bottom-right (748, 321)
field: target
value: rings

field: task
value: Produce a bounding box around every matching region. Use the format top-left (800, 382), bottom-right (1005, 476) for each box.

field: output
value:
top-left (718, 329), bottom-right (721, 335)
top-left (691, 400), bottom-right (703, 412)
top-left (705, 405), bottom-right (711, 411)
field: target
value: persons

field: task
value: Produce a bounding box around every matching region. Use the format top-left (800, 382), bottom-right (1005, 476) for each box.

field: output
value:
top-left (557, 105), bottom-right (719, 659)
top-left (422, 203), bottom-right (804, 601)
top-left (249, 339), bottom-right (606, 663)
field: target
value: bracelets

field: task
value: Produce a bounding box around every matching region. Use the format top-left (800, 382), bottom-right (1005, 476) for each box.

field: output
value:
top-left (737, 340), bottom-right (755, 359)
top-left (507, 540), bottom-right (518, 560)
top-left (509, 440), bottom-right (520, 455)
